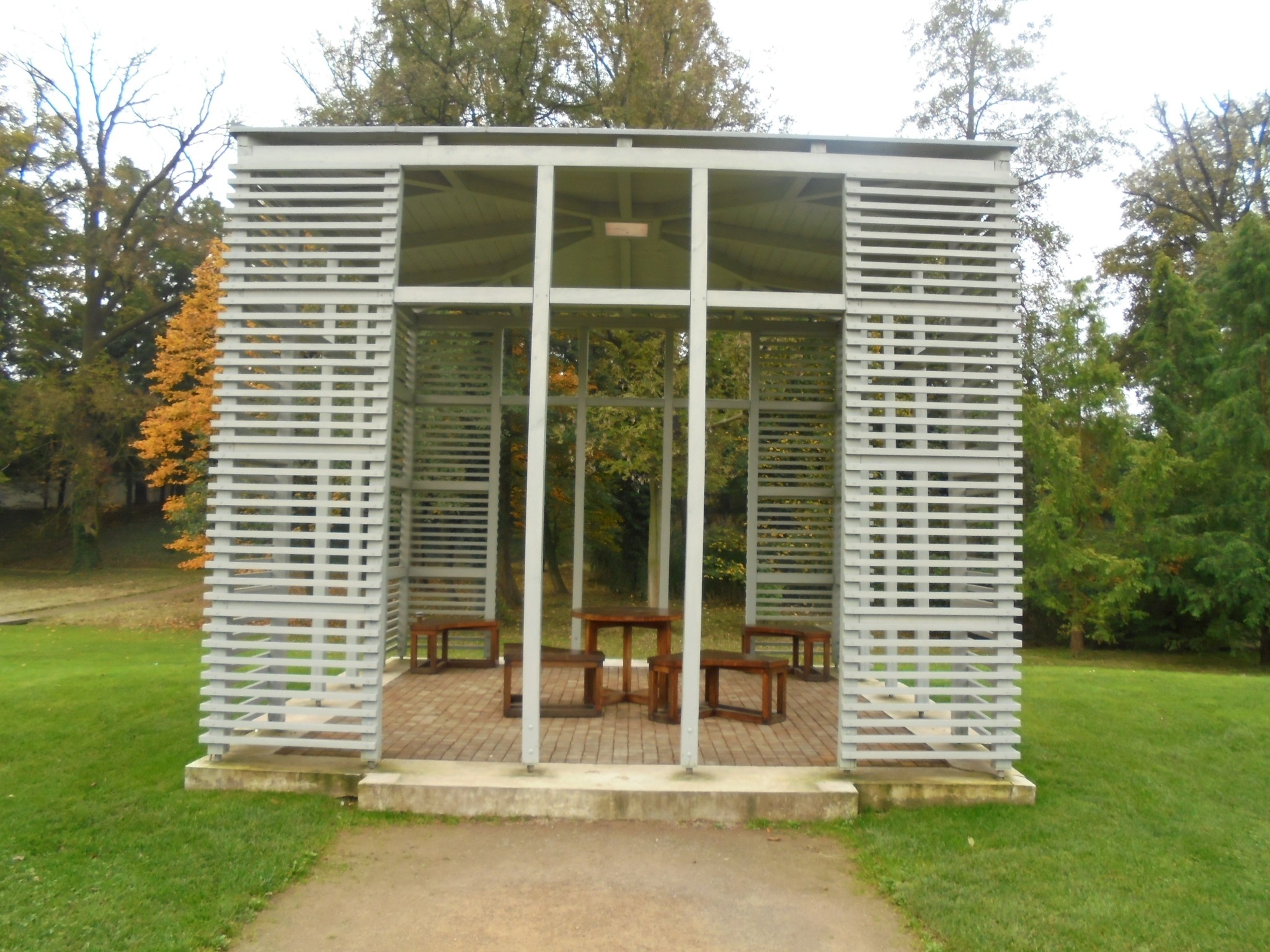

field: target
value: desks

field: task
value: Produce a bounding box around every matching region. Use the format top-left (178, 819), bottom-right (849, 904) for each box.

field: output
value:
top-left (572, 606), bottom-right (685, 709)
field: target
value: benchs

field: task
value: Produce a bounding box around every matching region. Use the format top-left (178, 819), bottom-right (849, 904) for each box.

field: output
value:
top-left (647, 647), bottom-right (788, 724)
top-left (740, 622), bottom-right (832, 682)
top-left (411, 614), bottom-right (501, 680)
top-left (505, 645), bottom-right (606, 718)
